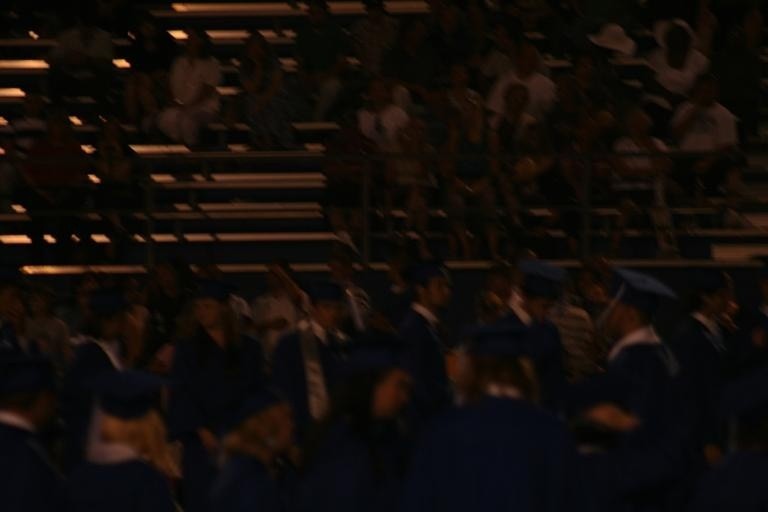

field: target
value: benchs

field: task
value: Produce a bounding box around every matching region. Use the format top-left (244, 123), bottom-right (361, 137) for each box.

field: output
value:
top-left (1, 4), bottom-right (342, 275)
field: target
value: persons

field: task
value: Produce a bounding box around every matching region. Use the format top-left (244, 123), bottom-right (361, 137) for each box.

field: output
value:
top-left (2, 2), bottom-right (765, 509)
